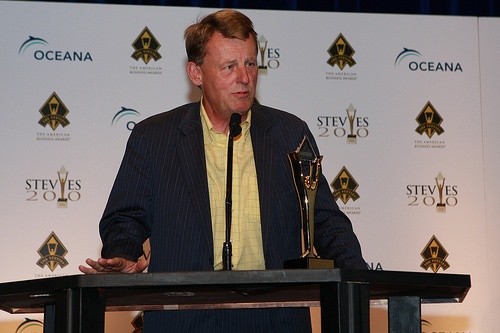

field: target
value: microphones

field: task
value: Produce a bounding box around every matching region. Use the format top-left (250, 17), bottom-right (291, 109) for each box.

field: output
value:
top-left (221, 113), bottom-right (242, 271)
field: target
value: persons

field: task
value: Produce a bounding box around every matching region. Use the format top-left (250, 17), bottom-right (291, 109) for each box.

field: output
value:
top-left (78, 7), bottom-right (375, 274)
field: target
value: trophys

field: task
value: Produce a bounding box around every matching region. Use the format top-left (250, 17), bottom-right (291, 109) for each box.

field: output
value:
top-left (282, 134), bottom-right (335, 272)
